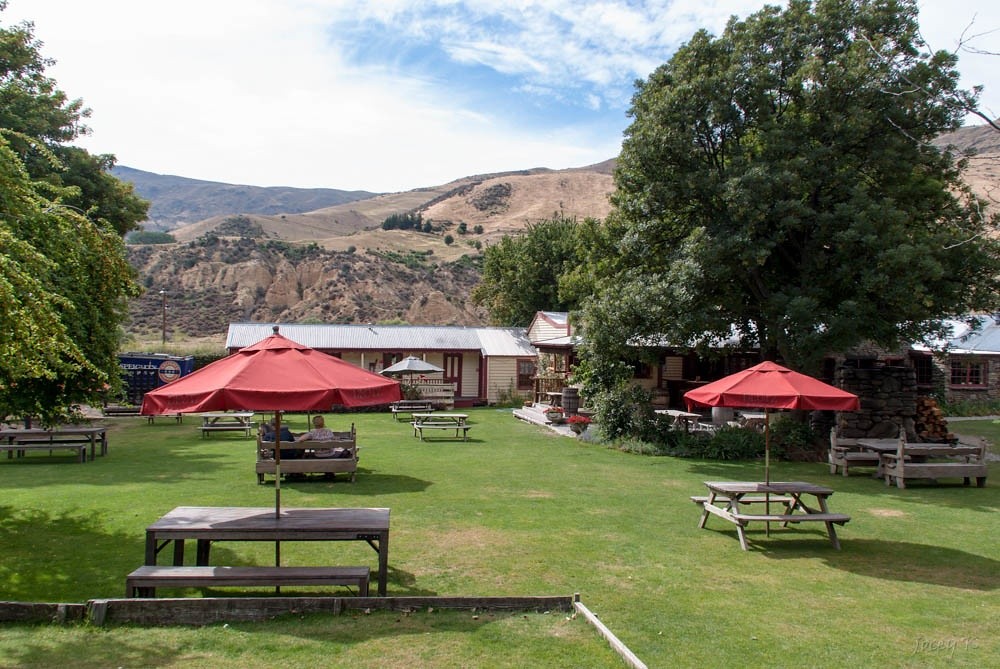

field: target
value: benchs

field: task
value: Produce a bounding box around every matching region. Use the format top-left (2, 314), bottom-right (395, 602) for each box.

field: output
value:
top-left (144, 415), bottom-right (182, 418)
top-left (409, 421), bottom-right (472, 431)
top-left (690, 495), bottom-right (852, 523)
top-left (126, 565), bottom-right (370, 598)
top-left (686, 419), bottom-right (745, 431)
top-left (197, 421), bottom-right (256, 431)
top-left (389, 405), bottom-right (435, 412)
top-left (255, 424), bottom-right (360, 485)
top-left (0, 437), bottom-right (108, 462)
top-left (827, 426), bottom-right (989, 490)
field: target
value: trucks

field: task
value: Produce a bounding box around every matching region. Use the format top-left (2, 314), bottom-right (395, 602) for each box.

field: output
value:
top-left (115, 352), bottom-right (195, 406)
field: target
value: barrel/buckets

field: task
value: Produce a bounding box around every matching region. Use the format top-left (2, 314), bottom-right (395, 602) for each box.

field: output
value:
top-left (711, 407), bottom-right (733, 426)
top-left (562, 387), bottom-right (579, 413)
top-left (651, 387), bottom-right (669, 410)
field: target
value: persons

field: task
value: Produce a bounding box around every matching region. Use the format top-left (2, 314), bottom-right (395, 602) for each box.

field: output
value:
top-left (295, 416), bottom-right (338, 480)
top-left (264, 416), bottom-right (307, 480)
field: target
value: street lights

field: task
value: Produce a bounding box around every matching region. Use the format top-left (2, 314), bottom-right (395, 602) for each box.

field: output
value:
top-left (160, 287), bottom-right (170, 344)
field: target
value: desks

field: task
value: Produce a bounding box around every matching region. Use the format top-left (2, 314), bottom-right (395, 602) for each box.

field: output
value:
top-left (412, 413), bottom-right (469, 442)
top-left (858, 439), bottom-right (952, 478)
top-left (200, 412), bottom-right (255, 439)
top-left (0, 428), bottom-right (106, 461)
top-left (698, 480), bottom-right (842, 552)
top-left (390, 401), bottom-right (433, 421)
top-left (546, 392), bottom-right (562, 406)
top-left (653, 409), bottom-right (702, 430)
top-left (738, 411), bottom-right (765, 431)
top-left (146, 506), bottom-right (392, 597)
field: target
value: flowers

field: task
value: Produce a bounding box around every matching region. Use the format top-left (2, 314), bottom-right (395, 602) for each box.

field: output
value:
top-left (542, 406), bottom-right (593, 424)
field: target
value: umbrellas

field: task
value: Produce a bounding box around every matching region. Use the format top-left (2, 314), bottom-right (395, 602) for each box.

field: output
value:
top-left (378, 355), bottom-right (447, 421)
top-left (141, 325), bottom-right (400, 593)
top-left (685, 361), bottom-right (858, 538)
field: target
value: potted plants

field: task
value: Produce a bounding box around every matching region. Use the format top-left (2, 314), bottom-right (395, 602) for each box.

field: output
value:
top-left (401, 383), bottom-right (422, 400)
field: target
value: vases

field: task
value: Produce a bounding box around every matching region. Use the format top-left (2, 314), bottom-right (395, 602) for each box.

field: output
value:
top-left (546, 412), bottom-right (563, 427)
top-left (570, 423), bottom-right (588, 435)
